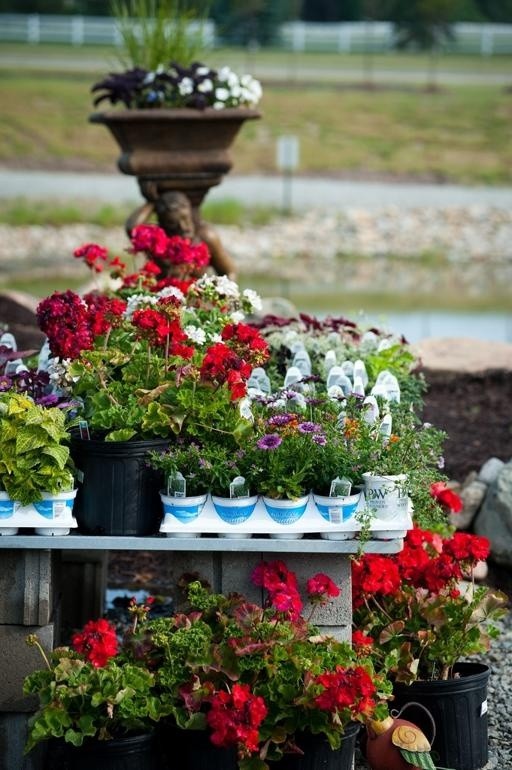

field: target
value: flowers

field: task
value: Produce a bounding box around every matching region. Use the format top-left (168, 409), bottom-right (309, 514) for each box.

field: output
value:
top-left (90, 59), bottom-right (263, 108)
top-left (73, 562), bottom-right (376, 755)
top-left (350, 478), bottom-right (495, 614)
top-left (37, 222), bottom-right (263, 326)
top-left (2, 319), bottom-right (449, 474)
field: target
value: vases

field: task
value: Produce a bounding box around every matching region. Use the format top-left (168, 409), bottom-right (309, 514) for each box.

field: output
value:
top-left (74, 433), bottom-right (163, 536)
top-left (385, 659), bottom-right (491, 769)
top-left (156, 472), bottom-right (410, 542)
top-left (89, 107), bottom-right (261, 278)
top-left (2, 482), bottom-right (77, 539)
